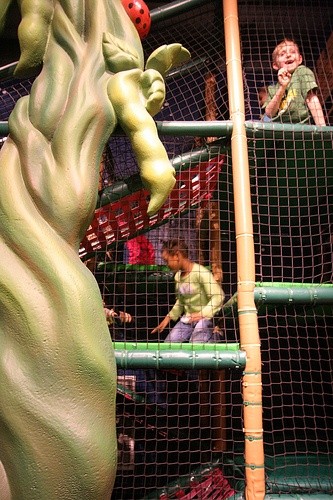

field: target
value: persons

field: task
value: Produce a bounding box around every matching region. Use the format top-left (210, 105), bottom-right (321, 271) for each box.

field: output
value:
top-left (149, 237), bottom-right (225, 343)
top-left (256, 86), bottom-right (273, 106)
top-left (260, 37), bottom-right (326, 127)
top-left (105, 306), bottom-right (131, 325)
